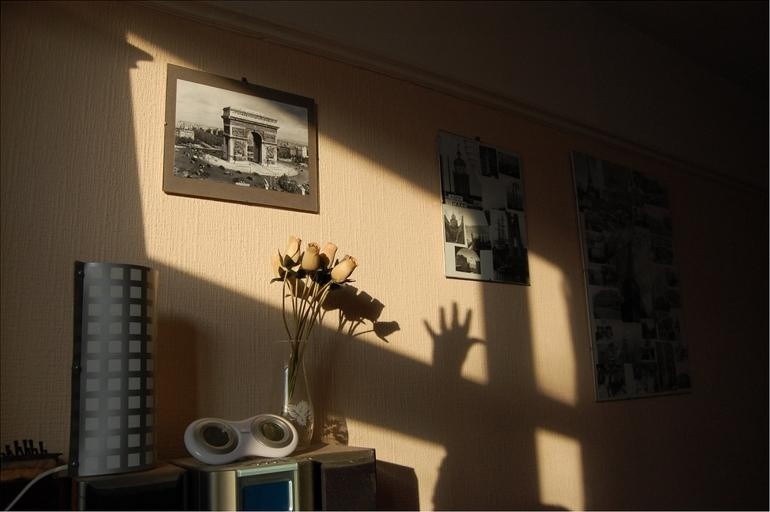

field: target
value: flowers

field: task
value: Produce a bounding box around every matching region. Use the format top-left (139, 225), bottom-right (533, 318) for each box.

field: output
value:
top-left (269, 237), bottom-right (357, 404)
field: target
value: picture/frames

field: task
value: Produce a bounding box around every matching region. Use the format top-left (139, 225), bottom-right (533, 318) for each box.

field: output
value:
top-left (163, 62), bottom-right (321, 214)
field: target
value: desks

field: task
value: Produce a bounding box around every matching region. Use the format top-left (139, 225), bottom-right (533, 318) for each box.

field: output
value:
top-left (0, 453), bottom-right (62, 512)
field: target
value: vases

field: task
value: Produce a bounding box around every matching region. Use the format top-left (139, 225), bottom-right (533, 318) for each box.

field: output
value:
top-left (270, 340), bottom-right (314, 453)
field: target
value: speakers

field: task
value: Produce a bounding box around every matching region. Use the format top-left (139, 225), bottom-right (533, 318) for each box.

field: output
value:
top-left (183, 413), bottom-right (299, 466)
